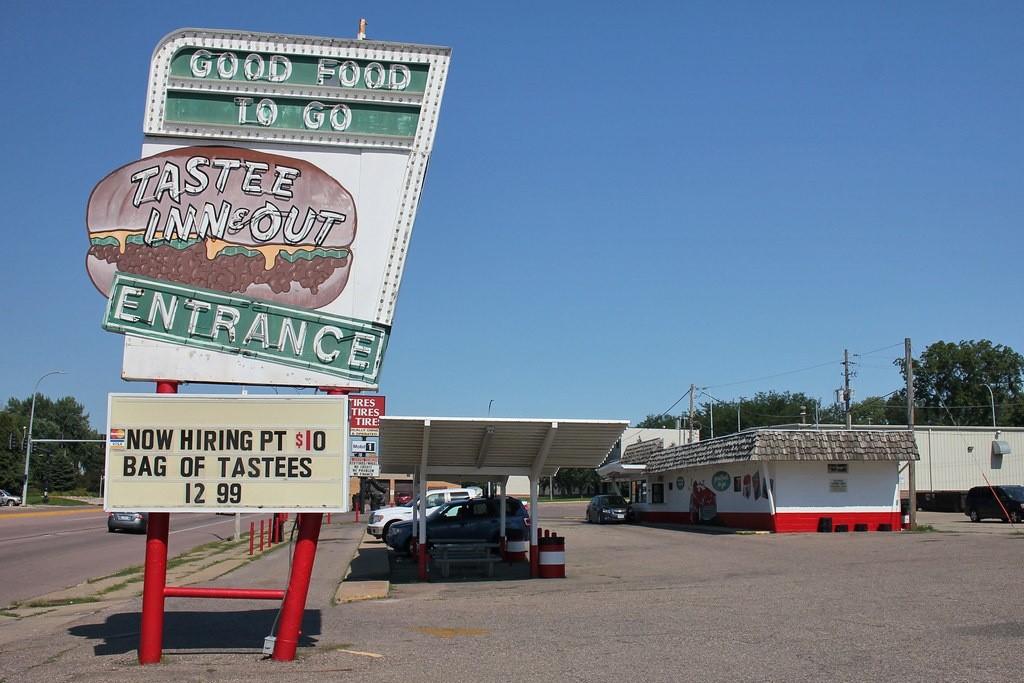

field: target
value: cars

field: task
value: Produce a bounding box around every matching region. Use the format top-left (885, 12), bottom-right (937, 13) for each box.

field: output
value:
top-left (107, 512), bottom-right (147, 534)
top-left (0, 490), bottom-right (22, 506)
top-left (585, 494), bottom-right (634, 524)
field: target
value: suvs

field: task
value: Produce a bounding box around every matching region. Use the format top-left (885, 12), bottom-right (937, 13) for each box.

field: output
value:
top-left (367, 488), bottom-right (481, 543)
top-left (965, 485), bottom-right (1024, 523)
top-left (387, 497), bottom-right (531, 552)
top-left (397, 493), bottom-right (411, 506)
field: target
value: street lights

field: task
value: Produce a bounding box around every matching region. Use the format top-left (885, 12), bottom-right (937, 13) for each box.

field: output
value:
top-left (703, 386), bottom-right (714, 438)
top-left (21, 371), bottom-right (67, 507)
top-left (738, 397), bottom-right (747, 432)
top-left (980, 383), bottom-right (995, 425)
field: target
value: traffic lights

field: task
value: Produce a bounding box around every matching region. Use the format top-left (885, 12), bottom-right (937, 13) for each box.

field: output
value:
top-left (33, 442), bottom-right (37, 452)
top-left (23, 439), bottom-right (28, 450)
top-left (11, 434), bottom-right (18, 449)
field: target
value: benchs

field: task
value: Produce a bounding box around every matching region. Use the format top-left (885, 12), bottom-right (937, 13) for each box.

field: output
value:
top-left (435, 558), bottom-right (503, 578)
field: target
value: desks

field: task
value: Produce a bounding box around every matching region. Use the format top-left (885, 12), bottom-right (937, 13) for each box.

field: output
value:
top-left (433, 543), bottom-right (501, 558)
top-left (428, 539), bottom-right (488, 542)
